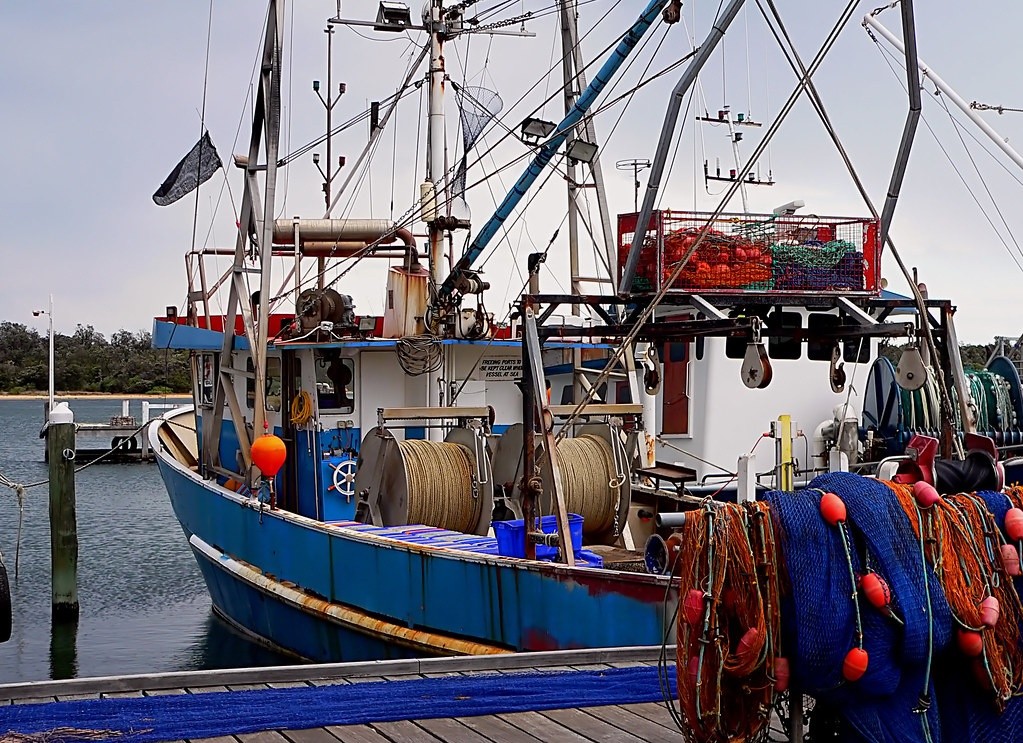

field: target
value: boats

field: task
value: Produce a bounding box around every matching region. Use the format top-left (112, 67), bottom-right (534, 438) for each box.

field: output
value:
top-left (144, 0), bottom-right (1023, 663)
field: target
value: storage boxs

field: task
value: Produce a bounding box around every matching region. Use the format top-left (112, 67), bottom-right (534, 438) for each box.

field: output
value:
top-left (325, 513), bottom-right (603, 569)
